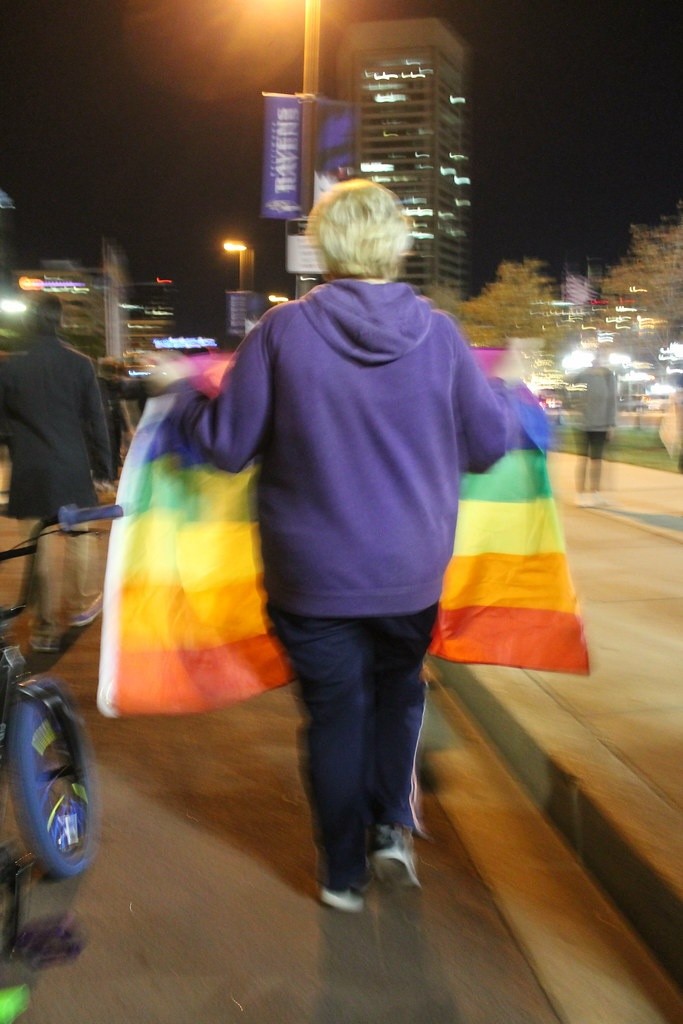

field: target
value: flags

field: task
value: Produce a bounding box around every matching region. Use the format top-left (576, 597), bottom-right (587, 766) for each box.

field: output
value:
top-left (259, 97), bottom-right (300, 217)
top-left (313, 101), bottom-right (356, 203)
top-left (97, 352), bottom-right (587, 717)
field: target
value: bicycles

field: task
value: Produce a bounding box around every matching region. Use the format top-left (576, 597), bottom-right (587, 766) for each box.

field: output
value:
top-left (1, 502), bottom-right (130, 969)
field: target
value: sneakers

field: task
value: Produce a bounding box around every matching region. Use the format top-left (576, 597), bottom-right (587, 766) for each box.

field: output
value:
top-left (319, 883), bottom-right (368, 914)
top-left (367, 821), bottom-right (422, 894)
top-left (66, 595), bottom-right (103, 628)
top-left (25, 630), bottom-right (63, 653)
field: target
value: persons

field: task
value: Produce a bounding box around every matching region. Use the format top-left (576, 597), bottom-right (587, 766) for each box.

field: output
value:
top-left (137, 178), bottom-right (515, 914)
top-left (0, 295), bottom-right (114, 650)
top-left (561, 348), bottom-right (617, 505)
